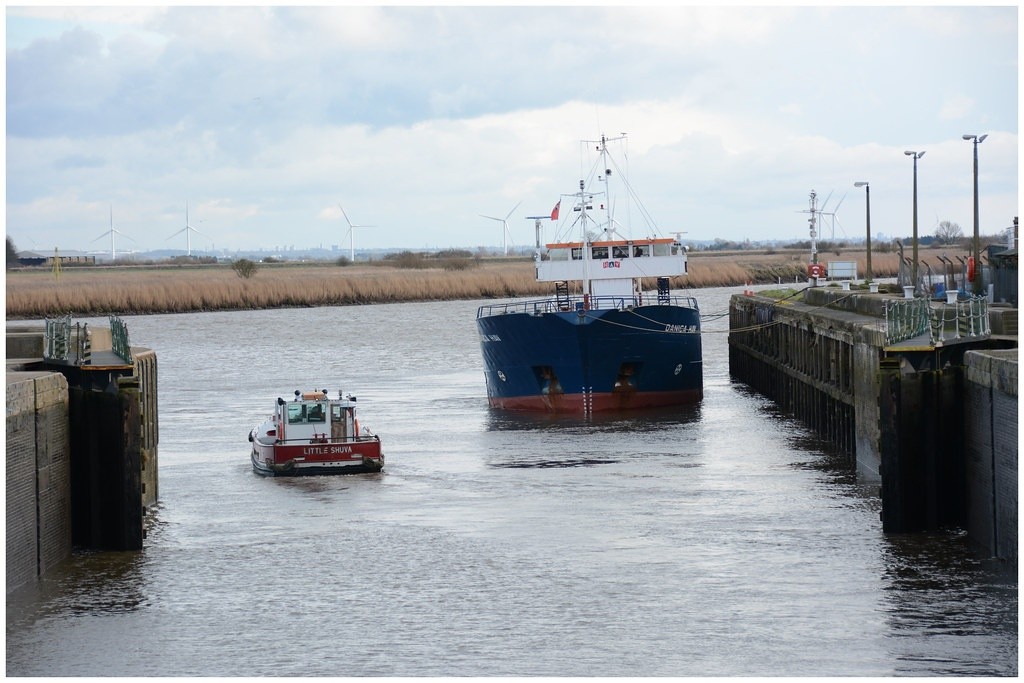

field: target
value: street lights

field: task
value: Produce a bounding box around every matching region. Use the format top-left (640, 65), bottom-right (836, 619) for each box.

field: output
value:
top-left (905, 150), bottom-right (926, 288)
top-left (963, 133), bottom-right (988, 293)
top-left (855, 181), bottom-right (875, 285)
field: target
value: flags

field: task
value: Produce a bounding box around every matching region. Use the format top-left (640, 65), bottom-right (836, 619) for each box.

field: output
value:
top-left (551, 201), bottom-right (561, 221)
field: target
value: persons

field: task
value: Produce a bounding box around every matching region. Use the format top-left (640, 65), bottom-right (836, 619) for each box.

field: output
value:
top-left (635, 247), bottom-right (641, 257)
top-left (617, 250), bottom-right (623, 258)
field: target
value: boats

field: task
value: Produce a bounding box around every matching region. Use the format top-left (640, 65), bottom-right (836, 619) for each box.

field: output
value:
top-left (478, 110), bottom-right (703, 422)
top-left (249, 389), bottom-right (384, 477)
